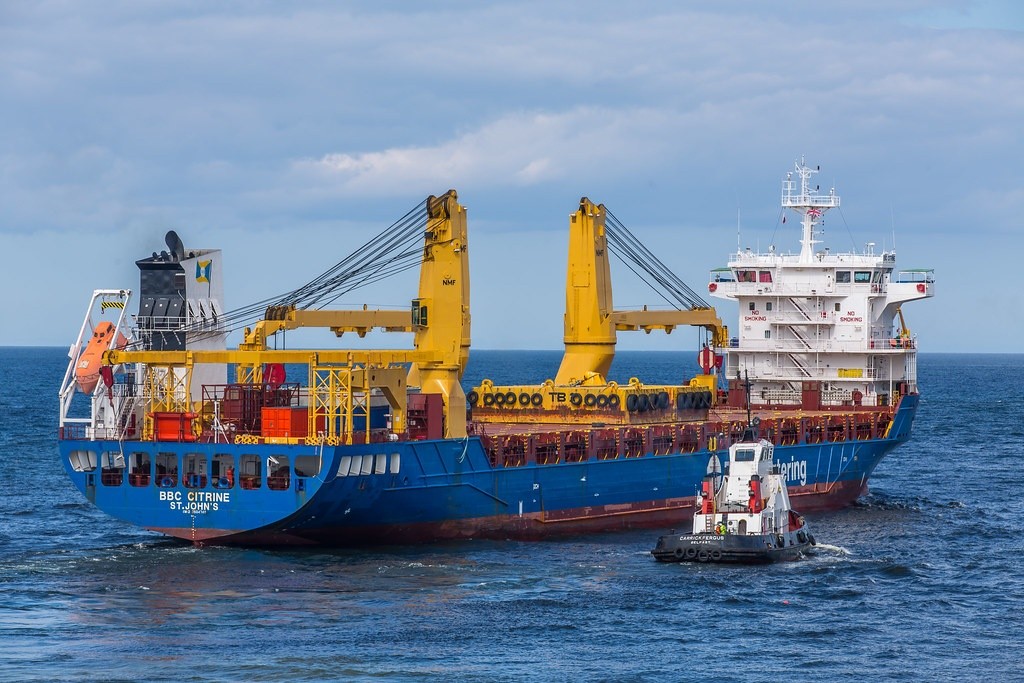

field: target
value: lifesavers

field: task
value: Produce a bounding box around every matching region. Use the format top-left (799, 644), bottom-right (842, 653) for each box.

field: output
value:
top-left (776, 529), bottom-right (817, 548)
top-left (571, 393), bottom-right (620, 407)
top-left (708, 283), bottom-right (718, 293)
top-left (677, 391), bottom-right (712, 409)
top-left (916, 284), bottom-right (925, 293)
top-left (627, 392), bottom-right (669, 412)
top-left (674, 545), bottom-right (721, 561)
top-left (466, 391), bottom-right (542, 406)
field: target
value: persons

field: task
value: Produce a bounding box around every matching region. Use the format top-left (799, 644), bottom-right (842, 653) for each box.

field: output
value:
top-left (227, 466), bottom-right (233, 488)
top-left (852, 388), bottom-right (862, 406)
top-left (718, 387), bottom-right (724, 397)
top-left (806, 429), bottom-right (810, 444)
top-left (727, 521), bottom-right (736, 535)
top-left (142, 461), bottom-right (150, 474)
top-left (716, 521), bottom-right (726, 536)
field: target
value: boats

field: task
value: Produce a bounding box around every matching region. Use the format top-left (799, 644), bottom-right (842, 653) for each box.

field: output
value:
top-left (54, 147), bottom-right (936, 547)
top-left (652, 439), bottom-right (818, 561)
